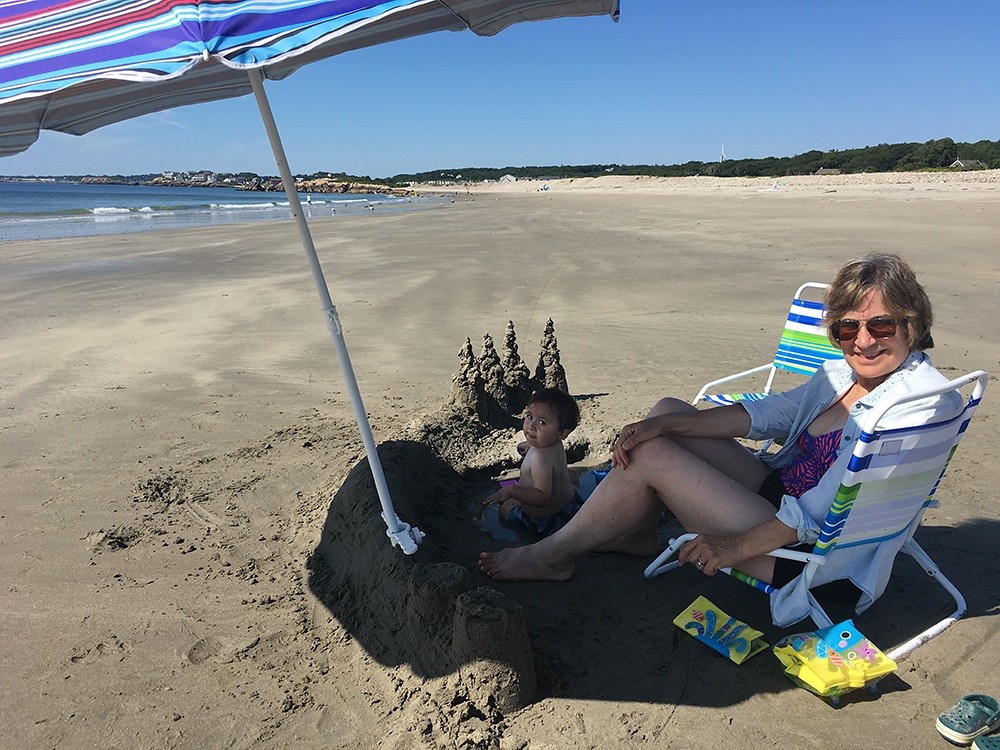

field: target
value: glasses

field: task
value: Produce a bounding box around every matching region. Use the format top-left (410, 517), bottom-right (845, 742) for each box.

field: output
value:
top-left (829, 314), bottom-right (905, 342)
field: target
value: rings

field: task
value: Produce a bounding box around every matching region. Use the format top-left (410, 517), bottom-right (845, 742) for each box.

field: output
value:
top-left (697, 556), bottom-right (706, 566)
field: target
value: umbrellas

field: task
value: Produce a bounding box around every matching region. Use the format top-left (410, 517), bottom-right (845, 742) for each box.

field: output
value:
top-left (0, 0), bottom-right (621, 555)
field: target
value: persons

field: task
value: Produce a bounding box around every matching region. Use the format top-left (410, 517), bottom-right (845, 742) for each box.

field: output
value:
top-left (483, 387), bottom-right (587, 538)
top-left (307, 193), bottom-right (311, 204)
top-left (477, 251), bottom-right (966, 632)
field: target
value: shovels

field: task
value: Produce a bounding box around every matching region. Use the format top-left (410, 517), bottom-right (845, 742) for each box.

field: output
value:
top-left (499, 479), bottom-right (518, 488)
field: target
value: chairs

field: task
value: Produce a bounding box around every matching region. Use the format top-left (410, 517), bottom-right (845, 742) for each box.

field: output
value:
top-left (645, 370), bottom-right (990, 659)
top-left (692, 282), bottom-right (846, 458)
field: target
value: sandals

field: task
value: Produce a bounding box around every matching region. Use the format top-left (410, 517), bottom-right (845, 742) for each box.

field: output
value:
top-left (935, 694), bottom-right (1000, 750)
